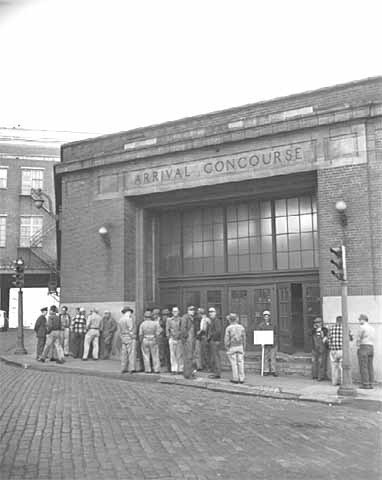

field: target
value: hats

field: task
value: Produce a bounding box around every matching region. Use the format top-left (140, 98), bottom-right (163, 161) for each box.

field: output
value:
top-left (208, 308), bottom-right (215, 311)
top-left (122, 307), bottom-right (133, 313)
top-left (144, 311), bottom-right (152, 317)
top-left (198, 307), bottom-right (205, 314)
top-left (41, 307), bottom-right (47, 311)
top-left (263, 311), bottom-right (271, 316)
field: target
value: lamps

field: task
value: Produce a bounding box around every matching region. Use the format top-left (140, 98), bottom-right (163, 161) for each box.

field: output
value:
top-left (99, 226), bottom-right (111, 248)
top-left (335, 200), bottom-right (348, 227)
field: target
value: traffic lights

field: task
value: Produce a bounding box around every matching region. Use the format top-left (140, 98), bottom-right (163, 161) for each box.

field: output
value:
top-left (329, 246), bottom-right (348, 281)
top-left (15, 266), bottom-right (24, 287)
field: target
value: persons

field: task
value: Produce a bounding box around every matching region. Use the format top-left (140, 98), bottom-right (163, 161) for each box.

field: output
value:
top-left (356, 314), bottom-right (375, 389)
top-left (34, 307), bottom-right (48, 360)
top-left (184, 306), bottom-right (203, 371)
top-left (251, 310), bottom-right (278, 376)
top-left (56, 306), bottom-right (72, 356)
top-left (37, 305), bottom-right (64, 364)
top-left (224, 312), bottom-right (247, 383)
top-left (138, 311), bottom-right (161, 373)
top-left (308, 316), bottom-right (328, 380)
top-left (119, 306), bottom-right (138, 372)
top-left (205, 307), bottom-right (223, 378)
top-left (71, 307), bottom-right (87, 358)
top-left (196, 308), bottom-right (212, 364)
top-left (152, 309), bottom-right (170, 370)
top-left (82, 308), bottom-right (102, 360)
top-left (328, 316), bottom-right (353, 385)
top-left (100, 310), bottom-right (117, 360)
top-left (165, 306), bottom-right (187, 374)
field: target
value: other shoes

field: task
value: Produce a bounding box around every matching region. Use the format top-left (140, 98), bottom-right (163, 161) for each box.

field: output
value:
top-left (264, 372), bottom-right (268, 376)
top-left (39, 353), bottom-right (71, 364)
top-left (313, 376), bottom-right (323, 381)
top-left (359, 385), bottom-right (373, 389)
top-left (231, 379), bottom-right (243, 384)
top-left (273, 372), bottom-right (278, 377)
